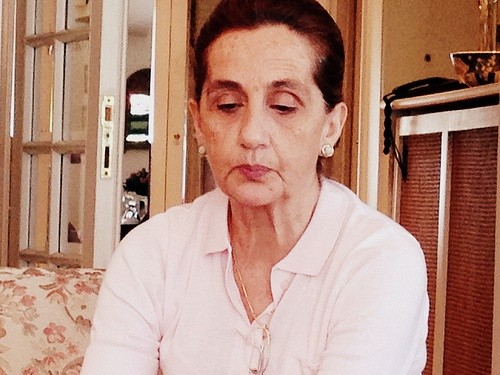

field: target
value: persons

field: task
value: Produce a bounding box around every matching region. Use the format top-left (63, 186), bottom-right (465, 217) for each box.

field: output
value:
top-left (79, 0), bottom-right (430, 375)
top-left (464, 56), bottom-right (496, 86)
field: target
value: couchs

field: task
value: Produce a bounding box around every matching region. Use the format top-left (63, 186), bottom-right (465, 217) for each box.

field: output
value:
top-left (0, 267), bottom-right (107, 375)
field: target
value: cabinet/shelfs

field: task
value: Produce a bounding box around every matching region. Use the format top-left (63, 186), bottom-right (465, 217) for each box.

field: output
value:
top-left (384, 84), bottom-right (500, 375)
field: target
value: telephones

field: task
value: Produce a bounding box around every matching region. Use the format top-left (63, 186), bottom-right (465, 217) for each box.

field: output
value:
top-left (392, 77), bottom-right (468, 100)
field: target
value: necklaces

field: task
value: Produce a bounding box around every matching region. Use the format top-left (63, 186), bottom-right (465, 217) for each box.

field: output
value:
top-left (228, 203), bottom-right (274, 343)
